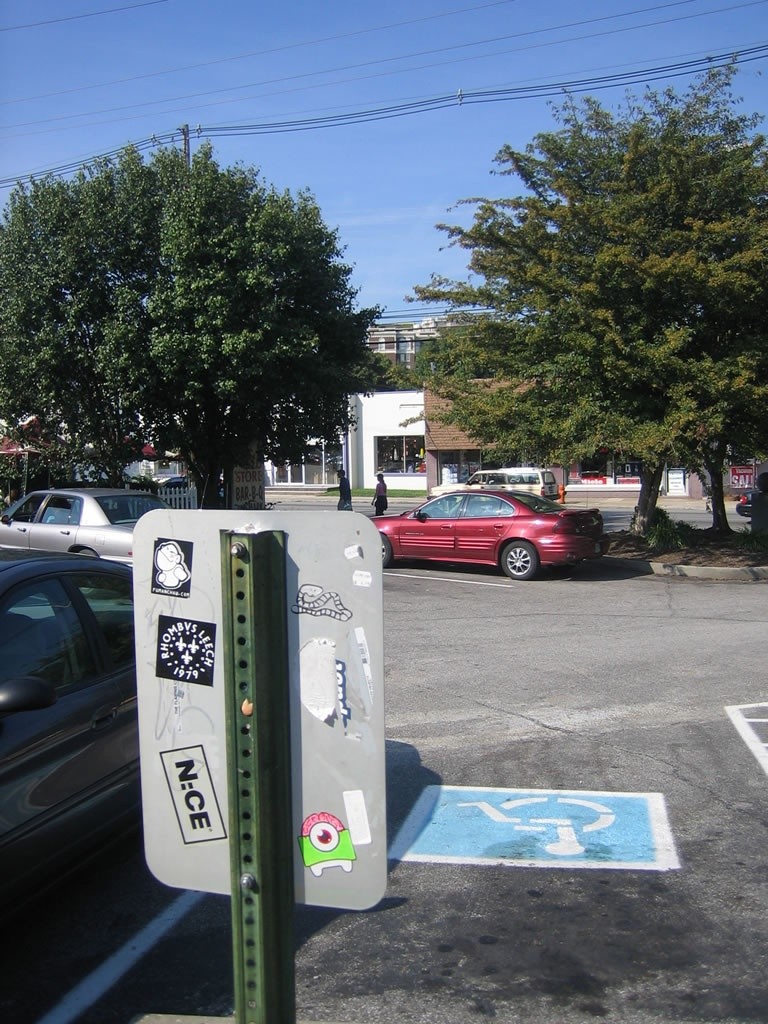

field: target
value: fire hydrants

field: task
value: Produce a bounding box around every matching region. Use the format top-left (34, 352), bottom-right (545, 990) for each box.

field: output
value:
top-left (558, 484), bottom-right (567, 504)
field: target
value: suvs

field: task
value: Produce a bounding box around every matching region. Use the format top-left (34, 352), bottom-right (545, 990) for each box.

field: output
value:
top-left (426, 467), bottom-right (561, 504)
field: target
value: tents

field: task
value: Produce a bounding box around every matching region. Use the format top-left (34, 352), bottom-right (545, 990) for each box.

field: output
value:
top-left (0, 415), bottom-right (180, 497)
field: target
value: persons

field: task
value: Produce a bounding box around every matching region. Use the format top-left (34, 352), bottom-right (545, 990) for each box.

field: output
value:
top-left (335, 470), bottom-right (353, 511)
top-left (371, 473), bottom-right (388, 516)
top-left (4, 489), bottom-right (16, 506)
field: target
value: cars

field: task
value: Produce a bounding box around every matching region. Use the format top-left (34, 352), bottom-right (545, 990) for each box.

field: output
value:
top-left (154, 474), bottom-right (223, 502)
top-left (369, 488), bottom-right (610, 581)
top-left (736, 490), bottom-right (762, 518)
top-left (0, 548), bottom-right (145, 916)
top-left (305, 447), bottom-right (343, 473)
top-left (0, 488), bottom-right (177, 566)
